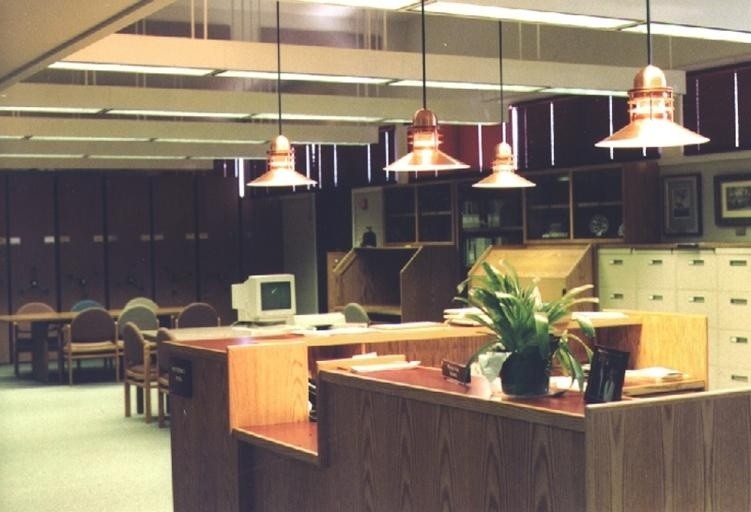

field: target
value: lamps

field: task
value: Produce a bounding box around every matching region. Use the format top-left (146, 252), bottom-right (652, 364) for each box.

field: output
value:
top-left (245, 0), bottom-right (319, 188)
top-left (471, 19), bottom-right (536, 189)
top-left (382, 0), bottom-right (471, 172)
top-left (594, 0), bottom-right (712, 149)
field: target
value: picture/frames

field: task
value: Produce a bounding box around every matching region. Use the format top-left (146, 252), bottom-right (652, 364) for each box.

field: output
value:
top-left (714, 173), bottom-right (751, 226)
top-left (661, 173), bottom-right (702, 237)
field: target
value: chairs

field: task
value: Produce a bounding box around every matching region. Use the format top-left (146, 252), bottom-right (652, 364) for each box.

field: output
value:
top-left (0, 297), bottom-right (371, 428)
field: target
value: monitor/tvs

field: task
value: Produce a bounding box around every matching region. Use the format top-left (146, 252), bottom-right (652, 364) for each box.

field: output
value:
top-left (231, 274), bottom-right (296, 325)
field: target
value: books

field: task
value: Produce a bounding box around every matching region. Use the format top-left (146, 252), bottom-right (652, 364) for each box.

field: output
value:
top-left (443, 306), bottom-right (489, 327)
top-left (557, 364), bottom-right (705, 396)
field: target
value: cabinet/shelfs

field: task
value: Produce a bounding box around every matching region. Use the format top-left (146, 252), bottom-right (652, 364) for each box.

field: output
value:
top-left (522, 161), bottom-right (661, 245)
top-left (383, 179), bottom-right (456, 247)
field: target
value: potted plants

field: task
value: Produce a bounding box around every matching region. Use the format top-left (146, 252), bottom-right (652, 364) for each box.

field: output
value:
top-left (451, 258), bottom-right (600, 398)
